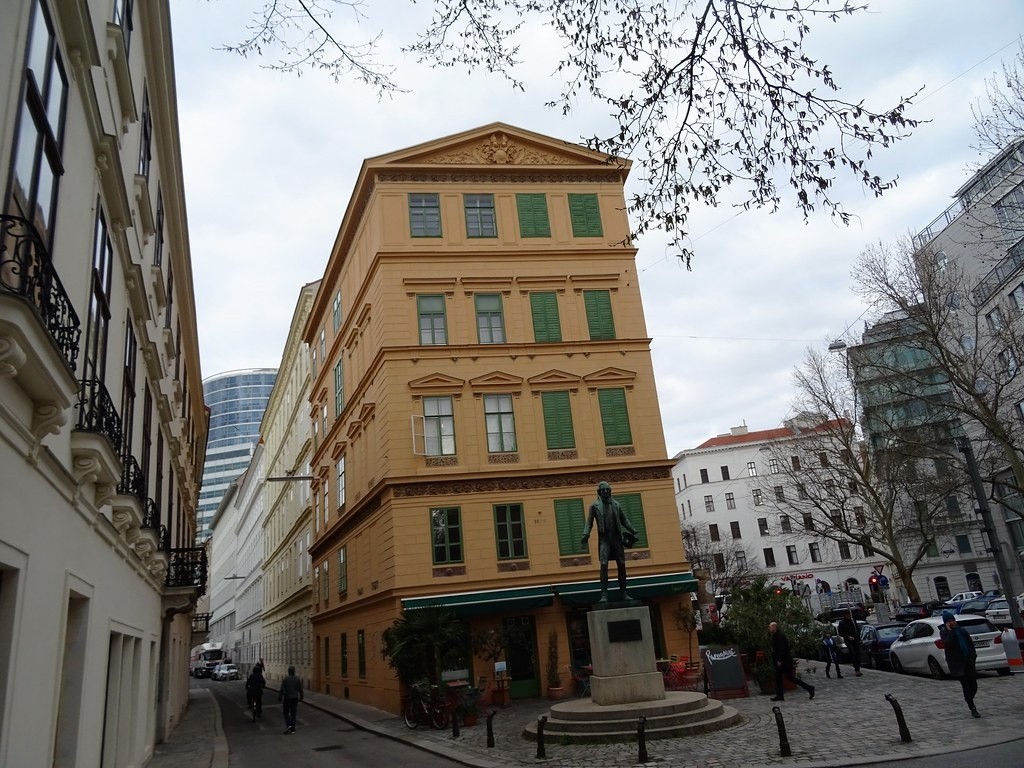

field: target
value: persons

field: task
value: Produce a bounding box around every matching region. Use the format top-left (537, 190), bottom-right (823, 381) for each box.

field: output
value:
top-left (838, 609), bottom-right (863, 677)
top-left (821, 632), bottom-right (844, 679)
top-left (768, 622), bottom-right (815, 701)
top-left (853, 603), bottom-right (870, 621)
top-left (938, 610), bottom-right (981, 718)
top-left (278, 666), bottom-right (303, 733)
top-left (580, 481), bottom-right (639, 604)
top-left (246, 653), bottom-right (266, 718)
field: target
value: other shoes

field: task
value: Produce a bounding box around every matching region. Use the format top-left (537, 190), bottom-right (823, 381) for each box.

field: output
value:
top-left (966, 699), bottom-right (979, 718)
top-left (824, 672), bottom-right (831, 678)
top-left (808, 686), bottom-right (814, 699)
top-left (771, 696), bottom-right (784, 701)
top-left (856, 672), bottom-right (860, 676)
top-left (283, 727), bottom-right (295, 734)
top-left (836, 674), bottom-right (842, 678)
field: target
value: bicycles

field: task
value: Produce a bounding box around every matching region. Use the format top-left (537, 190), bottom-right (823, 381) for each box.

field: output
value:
top-left (404, 684), bottom-right (452, 730)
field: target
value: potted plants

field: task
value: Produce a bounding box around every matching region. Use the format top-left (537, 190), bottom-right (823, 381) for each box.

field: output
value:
top-left (545, 630), bottom-right (564, 699)
top-left (472, 622), bottom-right (521, 708)
top-left (381, 598), bottom-right (469, 726)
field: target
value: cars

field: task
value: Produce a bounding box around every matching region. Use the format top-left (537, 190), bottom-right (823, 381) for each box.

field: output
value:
top-left (212, 664), bottom-right (240, 681)
top-left (889, 614), bottom-right (1015, 679)
top-left (831, 590), bottom-right (1024, 670)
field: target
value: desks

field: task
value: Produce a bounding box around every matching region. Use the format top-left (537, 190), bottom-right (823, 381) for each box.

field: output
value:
top-left (481, 676), bottom-right (512, 708)
top-left (656, 659), bottom-right (675, 691)
top-left (578, 666), bottom-right (593, 672)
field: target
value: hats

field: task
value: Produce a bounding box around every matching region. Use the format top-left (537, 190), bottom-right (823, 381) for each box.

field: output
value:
top-left (943, 611), bottom-right (953, 619)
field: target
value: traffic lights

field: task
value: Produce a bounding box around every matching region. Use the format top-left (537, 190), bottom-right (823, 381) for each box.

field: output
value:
top-left (871, 579), bottom-right (876, 583)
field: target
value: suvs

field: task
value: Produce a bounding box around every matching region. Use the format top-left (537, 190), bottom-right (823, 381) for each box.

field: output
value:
top-left (813, 602), bottom-right (870, 624)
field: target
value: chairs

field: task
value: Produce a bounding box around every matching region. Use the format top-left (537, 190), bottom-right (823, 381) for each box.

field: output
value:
top-left (568, 665), bottom-right (591, 698)
top-left (662, 655), bottom-right (704, 692)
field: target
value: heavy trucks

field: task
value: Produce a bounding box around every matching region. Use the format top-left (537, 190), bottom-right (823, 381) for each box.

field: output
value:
top-left (190, 642), bottom-right (227, 679)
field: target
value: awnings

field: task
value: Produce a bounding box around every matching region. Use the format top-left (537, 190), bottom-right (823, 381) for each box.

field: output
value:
top-left (396, 568), bottom-right (699, 624)
